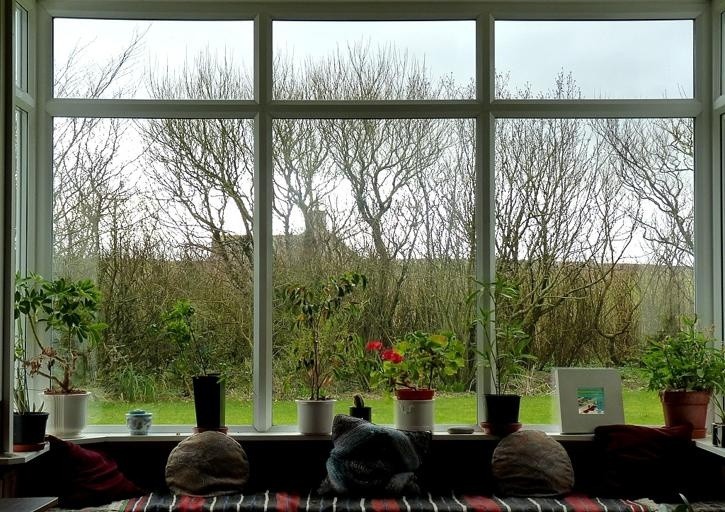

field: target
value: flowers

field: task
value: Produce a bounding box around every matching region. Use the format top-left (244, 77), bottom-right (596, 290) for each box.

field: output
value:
top-left (366, 326), bottom-right (466, 388)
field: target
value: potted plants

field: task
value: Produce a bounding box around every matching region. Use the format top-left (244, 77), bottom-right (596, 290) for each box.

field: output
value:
top-left (274, 272), bottom-right (376, 434)
top-left (348, 392), bottom-right (373, 423)
top-left (13, 271), bottom-right (110, 436)
top-left (464, 271), bottom-right (539, 435)
top-left (627, 309), bottom-right (725, 447)
top-left (13, 334), bottom-right (50, 444)
top-left (118, 360), bottom-right (161, 434)
top-left (141, 298), bottom-right (230, 435)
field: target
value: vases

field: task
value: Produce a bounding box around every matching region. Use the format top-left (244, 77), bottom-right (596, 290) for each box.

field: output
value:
top-left (393, 385), bottom-right (436, 432)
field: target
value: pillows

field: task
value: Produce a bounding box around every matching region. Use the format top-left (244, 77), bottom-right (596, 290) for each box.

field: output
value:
top-left (164, 429), bottom-right (252, 499)
top-left (488, 430), bottom-right (576, 501)
top-left (42, 433), bottom-right (153, 510)
top-left (316, 413), bottom-right (432, 502)
top-left (594, 422), bottom-right (701, 499)
